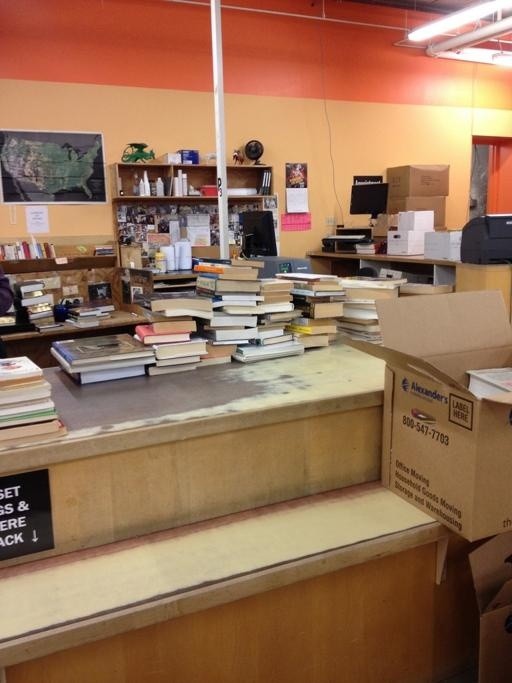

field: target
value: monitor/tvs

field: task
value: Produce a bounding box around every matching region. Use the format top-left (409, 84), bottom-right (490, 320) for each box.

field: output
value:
top-left (350, 184), bottom-right (389, 227)
top-left (239, 210), bottom-right (278, 258)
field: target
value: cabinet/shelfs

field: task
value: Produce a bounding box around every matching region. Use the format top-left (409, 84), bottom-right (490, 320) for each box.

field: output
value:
top-left (1, 235), bottom-right (149, 344)
top-left (111, 159), bottom-right (279, 289)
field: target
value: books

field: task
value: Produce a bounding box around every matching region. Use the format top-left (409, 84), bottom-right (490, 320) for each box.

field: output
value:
top-left (127, 167), bottom-right (191, 198)
top-left (1, 237), bottom-right (409, 385)
top-left (1, 353), bottom-right (69, 450)
top-left (465, 368), bottom-right (512, 401)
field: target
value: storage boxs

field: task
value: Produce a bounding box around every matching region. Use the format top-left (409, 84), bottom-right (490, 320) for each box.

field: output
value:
top-left (383, 163), bottom-right (451, 229)
top-left (331, 286), bottom-right (509, 546)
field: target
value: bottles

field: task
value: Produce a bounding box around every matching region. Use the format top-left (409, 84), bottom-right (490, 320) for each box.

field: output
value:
top-left (130, 171), bottom-right (165, 196)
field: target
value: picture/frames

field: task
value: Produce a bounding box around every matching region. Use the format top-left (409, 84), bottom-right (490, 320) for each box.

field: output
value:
top-left (0, 128), bottom-right (111, 204)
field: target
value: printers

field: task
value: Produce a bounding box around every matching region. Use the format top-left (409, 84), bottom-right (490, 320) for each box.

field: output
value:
top-left (460, 214), bottom-right (512, 265)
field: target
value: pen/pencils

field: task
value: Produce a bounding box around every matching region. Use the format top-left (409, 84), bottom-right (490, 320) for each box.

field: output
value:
top-left (60, 297), bottom-right (65, 306)
top-left (131, 312), bottom-right (138, 317)
